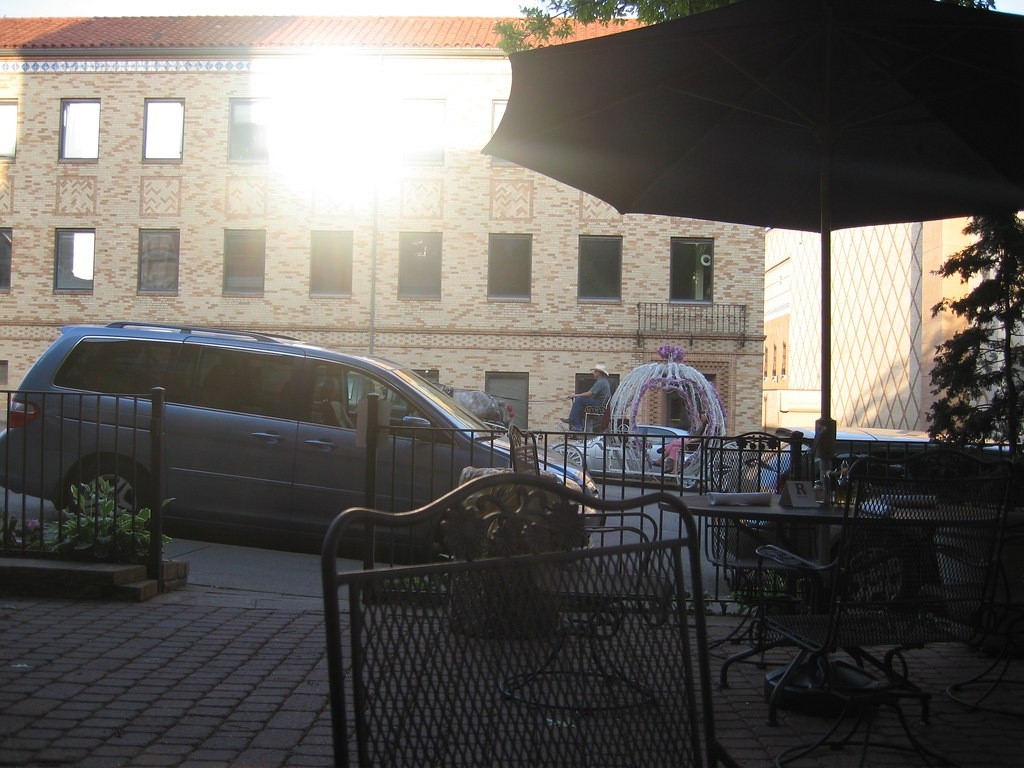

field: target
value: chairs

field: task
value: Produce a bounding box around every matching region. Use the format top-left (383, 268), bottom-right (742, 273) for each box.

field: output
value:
top-left (711, 432), bottom-right (1024, 768)
top-left (320, 473), bottom-right (745, 768)
top-left (498, 424), bottom-right (673, 710)
top-left (322, 376), bottom-right (354, 429)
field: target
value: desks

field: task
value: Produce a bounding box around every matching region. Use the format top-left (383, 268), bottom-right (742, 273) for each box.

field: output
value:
top-left (660, 496), bottom-right (1024, 727)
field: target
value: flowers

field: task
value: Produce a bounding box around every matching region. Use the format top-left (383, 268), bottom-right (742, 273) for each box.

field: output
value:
top-left (657, 345), bottom-right (684, 361)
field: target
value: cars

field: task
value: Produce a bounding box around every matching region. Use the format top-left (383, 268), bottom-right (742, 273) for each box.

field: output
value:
top-left (549, 424), bottom-right (711, 492)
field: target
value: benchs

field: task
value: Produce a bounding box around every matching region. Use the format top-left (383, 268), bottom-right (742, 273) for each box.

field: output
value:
top-left (569, 393), bottom-right (612, 439)
top-left (686, 423), bottom-right (721, 450)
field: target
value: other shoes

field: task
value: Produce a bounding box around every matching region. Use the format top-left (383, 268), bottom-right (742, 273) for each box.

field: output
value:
top-left (561, 417), bottom-right (575, 426)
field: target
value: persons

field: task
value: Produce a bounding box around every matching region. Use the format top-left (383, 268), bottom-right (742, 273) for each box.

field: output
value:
top-left (559, 365), bottom-right (610, 426)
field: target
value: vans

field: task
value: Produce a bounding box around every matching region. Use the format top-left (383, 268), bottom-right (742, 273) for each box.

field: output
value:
top-left (716, 425), bottom-right (1024, 564)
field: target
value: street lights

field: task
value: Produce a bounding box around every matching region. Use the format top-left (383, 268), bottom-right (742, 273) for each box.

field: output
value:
top-left (337, 67), bottom-right (407, 360)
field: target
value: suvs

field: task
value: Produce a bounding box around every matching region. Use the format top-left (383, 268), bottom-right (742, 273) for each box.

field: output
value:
top-left (2, 320), bottom-right (606, 563)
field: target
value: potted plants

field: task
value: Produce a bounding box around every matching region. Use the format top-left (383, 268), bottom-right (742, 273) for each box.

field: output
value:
top-left (381, 577), bottom-right (449, 608)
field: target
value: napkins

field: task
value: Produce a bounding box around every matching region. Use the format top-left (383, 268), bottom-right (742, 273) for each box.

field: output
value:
top-left (881, 493), bottom-right (936, 508)
top-left (707, 492), bottom-right (771, 506)
top-left (863, 502), bottom-right (894, 517)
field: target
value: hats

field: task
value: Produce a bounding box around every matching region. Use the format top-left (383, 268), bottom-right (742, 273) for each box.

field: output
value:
top-left (590, 364), bottom-right (608, 376)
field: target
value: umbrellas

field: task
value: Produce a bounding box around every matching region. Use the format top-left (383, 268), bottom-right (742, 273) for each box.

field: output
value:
top-left (476, 0), bottom-right (1024, 603)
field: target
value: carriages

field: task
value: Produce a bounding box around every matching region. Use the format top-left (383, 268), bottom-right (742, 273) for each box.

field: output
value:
top-left (379, 345), bottom-right (727, 491)
